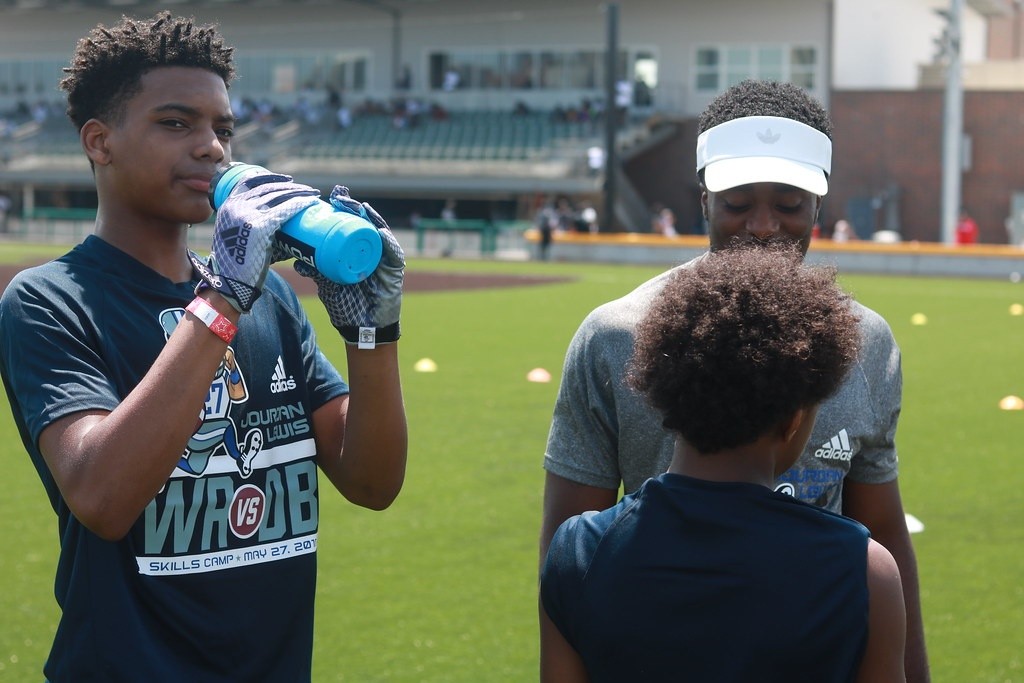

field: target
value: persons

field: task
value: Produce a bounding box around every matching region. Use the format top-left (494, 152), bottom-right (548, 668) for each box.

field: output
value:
top-left (539, 243), bottom-right (911, 683)
top-left (540, 81), bottom-right (929, 683)
top-left (0, 13), bottom-right (409, 683)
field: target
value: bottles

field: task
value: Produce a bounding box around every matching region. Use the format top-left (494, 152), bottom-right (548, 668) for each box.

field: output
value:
top-left (205, 160), bottom-right (382, 284)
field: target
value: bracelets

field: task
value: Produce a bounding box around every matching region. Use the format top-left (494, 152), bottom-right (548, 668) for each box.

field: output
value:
top-left (185, 295), bottom-right (238, 344)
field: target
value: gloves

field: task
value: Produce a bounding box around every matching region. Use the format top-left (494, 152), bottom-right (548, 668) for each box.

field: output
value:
top-left (293, 185), bottom-right (406, 349)
top-left (186, 173), bottom-right (321, 314)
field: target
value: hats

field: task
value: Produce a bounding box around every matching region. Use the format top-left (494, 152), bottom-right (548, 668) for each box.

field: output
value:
top-left (696, 116), bottom-right (832, 195)
